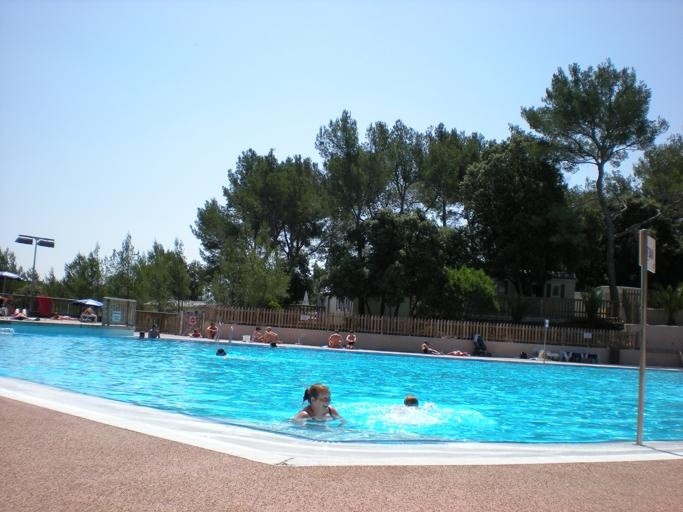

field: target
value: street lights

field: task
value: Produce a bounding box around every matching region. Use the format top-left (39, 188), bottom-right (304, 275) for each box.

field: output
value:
top-left (13, 234), bottom-right (54, 318)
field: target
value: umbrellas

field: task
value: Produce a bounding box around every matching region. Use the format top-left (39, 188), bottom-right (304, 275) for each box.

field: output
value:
top-left (72, 298), bottom-right (103, 307)
top-left (0, 270), bottom-right (23, 294)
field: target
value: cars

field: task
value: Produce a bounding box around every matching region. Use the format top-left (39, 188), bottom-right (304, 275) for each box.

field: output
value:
top-left (444, 308), bottom-right (626, 344)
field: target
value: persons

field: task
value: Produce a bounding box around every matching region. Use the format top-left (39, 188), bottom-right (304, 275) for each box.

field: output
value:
top-left (138, 332), bottom-right (146, 340)
top-left (404, 397), bottom-right (418, 408)
top-left (149, 324), bottom-right (160, 339)
top-left (422, 340), bottom-right (440, 354)
top-left (182, 310), bottom-right (281, 356)
top-left (448, 350), bottom-right (467, 356)
top-left (84, 306), bottom-right (96, 316)
top-left (294, 384), bottom-right (348, 432)
top-left (330, 330), bottom-right (344, 348)
top-left (346, 330), bottom-right (356, 348)
